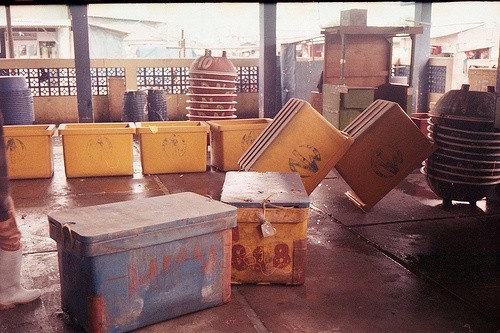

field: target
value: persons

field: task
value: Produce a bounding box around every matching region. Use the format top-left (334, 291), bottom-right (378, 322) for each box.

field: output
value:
top-left (0, 112), bottom-right (42, 310)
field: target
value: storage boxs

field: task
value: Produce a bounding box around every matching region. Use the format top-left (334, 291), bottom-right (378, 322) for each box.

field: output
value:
top-left (340, 9), bottom-right (368, 26)
top-left (342, 87), bottom-right (378, 109)
top-left (2, 125), bottom-right (56, 180)
top-left (207, 118), bottom-right (273, 171)
top-left (58, 122), bottom-right (137, 178)
top-left (136, 121), bottom-right (210, 175)
top-left (332, 99), bottom-right (438, 214)
top-left (220, 171), bottom-right (310, 286)
top-left (237, 98), bottom-right (354, 197)
top-left (47, 191), bottom-right (237, 333)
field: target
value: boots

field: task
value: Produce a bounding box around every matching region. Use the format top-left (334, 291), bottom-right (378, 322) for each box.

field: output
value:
top-left (1, 247), bottom-right (41, 310)
